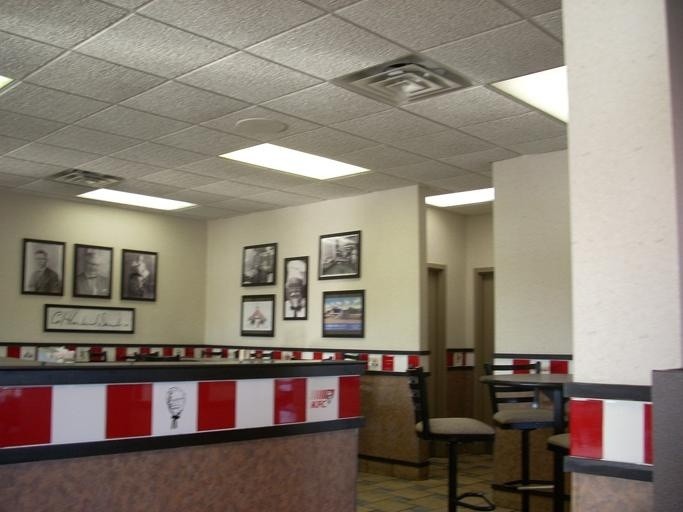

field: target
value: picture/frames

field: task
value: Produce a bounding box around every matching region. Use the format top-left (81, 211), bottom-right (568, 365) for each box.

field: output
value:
top-left (20, 237), bottom-right (64, 296)
top-left (240, 293), bottom-right (275, 337)
top-left (121, 248), bottom-right (157, 302)
top-left (71, 243), bottom-right (113, 299)
top-left (241, 243), bottom-right (277, 286)
top-left (317, 229), bottom-right (360, 280)
top-left (42, 303), bottom-right (135, 334)
top-left (321, 288), bottom-right (365, 338)
top-left (283, 255), bottom-right (308, 321)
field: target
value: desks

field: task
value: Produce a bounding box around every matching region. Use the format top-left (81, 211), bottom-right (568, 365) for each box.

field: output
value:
top-left (476, 371), bottom-right (571, 511)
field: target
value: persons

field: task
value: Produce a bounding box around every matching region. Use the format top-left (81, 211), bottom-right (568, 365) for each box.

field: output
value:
top-left (28, 251), bottom-right (61, 293)
top-left (166, 387), bottom-right (186, 431)
top-left (75, 253), bottom-right (109, 296)
top-left (285, 278), bottom-right (305, 317)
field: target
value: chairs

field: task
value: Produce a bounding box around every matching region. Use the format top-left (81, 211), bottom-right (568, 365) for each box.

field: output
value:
top-left (84, 348), bottom-right (361, 361)
top-left (543, 430), bottom-right (572, 456)
top-left (480, 360), bottom-right (560, 512)
top-left (403, 360), bottom-right (499, 512)
